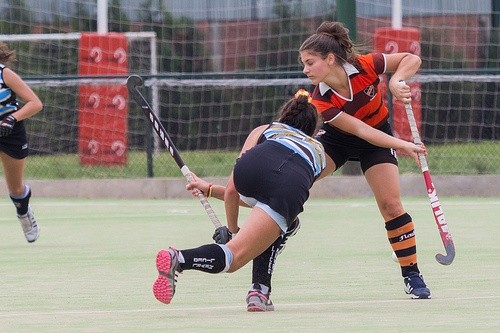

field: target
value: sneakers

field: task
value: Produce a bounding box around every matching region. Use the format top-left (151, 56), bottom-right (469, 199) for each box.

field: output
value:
top-left (245, 282), bottom-right (274, 312)
top-left (401, 270), bottom-right (431, 299)
top-left (16, 205), bottom-right (40, 243)
top-left (276, 216), bottom-right (300, 256)
top-left (153, 245), bottom-right (184, 305)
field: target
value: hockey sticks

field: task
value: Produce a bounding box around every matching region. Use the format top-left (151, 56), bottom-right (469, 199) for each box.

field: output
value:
top-left (127, 75), bottom-right (223, 230)
top-left (398, 80), bottom-right (456, 265)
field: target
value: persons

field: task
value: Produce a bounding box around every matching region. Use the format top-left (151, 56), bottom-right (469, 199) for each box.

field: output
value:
top-left (153, 95), bottom-right (327, 312)
top-left (0, 41), bottom-right (43, 242)
top-left (298, 20), bottom-right (431, 300)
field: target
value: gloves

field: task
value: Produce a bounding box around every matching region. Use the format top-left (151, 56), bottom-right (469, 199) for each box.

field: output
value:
top-left (0, 114), bottom-right (17, 137)
top-left (213, 224), bottom-right (241, 244)
